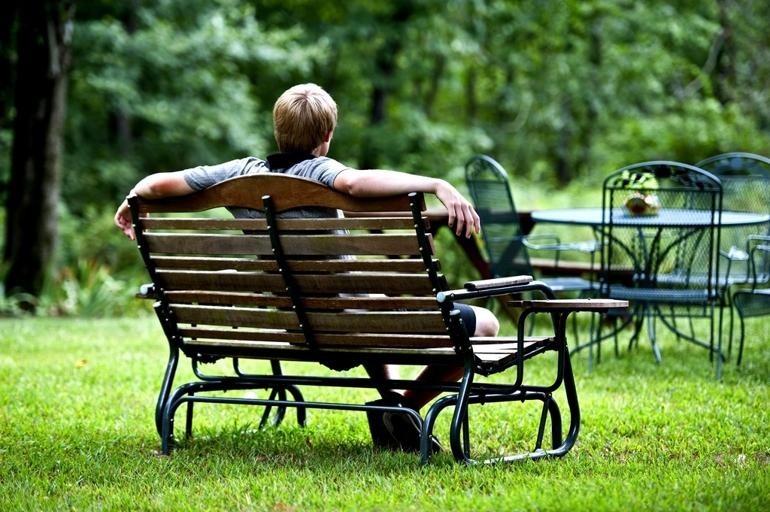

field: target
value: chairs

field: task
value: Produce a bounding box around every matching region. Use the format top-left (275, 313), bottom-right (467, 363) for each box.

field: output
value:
top-left (724, 234), bottom-right (770, 367)
top-left (464, 153), bottom-right (599, 376)
top-left (667, 149), bottom-right (770, 366)
top-left (591, 158), bottom-right (725, 373)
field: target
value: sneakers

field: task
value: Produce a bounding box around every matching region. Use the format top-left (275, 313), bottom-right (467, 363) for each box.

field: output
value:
top-left (365, 397), bottom-right (398, 450)
top-left (382, 410), bottom-right (444, 454)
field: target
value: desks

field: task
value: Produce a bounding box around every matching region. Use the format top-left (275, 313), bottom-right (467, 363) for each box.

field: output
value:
top-left (343, 204), bottom-right (531, 334)
top-left (528, 204), bottom-right (770, 358)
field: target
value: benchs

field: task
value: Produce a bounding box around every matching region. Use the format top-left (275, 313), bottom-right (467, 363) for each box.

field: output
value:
top-left (123, 177), bottom-right (632, 468)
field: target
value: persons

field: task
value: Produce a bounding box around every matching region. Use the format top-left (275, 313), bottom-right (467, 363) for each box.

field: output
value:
top-left (112, 81), bottom-right (501, 457)
